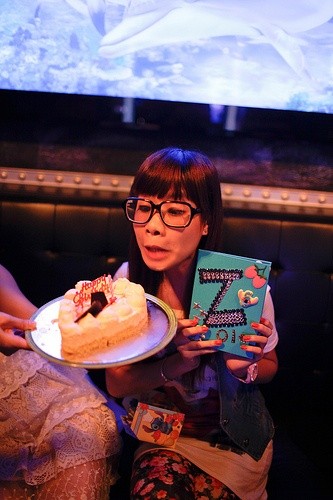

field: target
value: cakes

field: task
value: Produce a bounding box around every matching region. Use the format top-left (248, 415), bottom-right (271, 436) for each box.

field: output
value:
top-left (57, 262), bottom-right (149, 361)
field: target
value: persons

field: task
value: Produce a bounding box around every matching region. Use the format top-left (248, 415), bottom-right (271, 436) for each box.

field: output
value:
top-left (0, 265), bottom-right (129, 500)
top-left (104, 147), bottom-right (280, 500)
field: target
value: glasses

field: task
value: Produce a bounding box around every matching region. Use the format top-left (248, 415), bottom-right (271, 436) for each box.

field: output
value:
top-left (120, 197), bottom-right (203, 228)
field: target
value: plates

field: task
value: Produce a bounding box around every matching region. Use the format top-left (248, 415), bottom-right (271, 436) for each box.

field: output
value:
top-left (25, 293), bottom-right (178, 369)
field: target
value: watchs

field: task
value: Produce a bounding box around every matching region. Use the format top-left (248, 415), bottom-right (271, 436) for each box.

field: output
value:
top-left (229, 363), bottom-right (258, 384)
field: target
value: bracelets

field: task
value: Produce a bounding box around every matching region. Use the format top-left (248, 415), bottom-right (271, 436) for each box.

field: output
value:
top-left (163, 357), bottom-right (178, 381)
